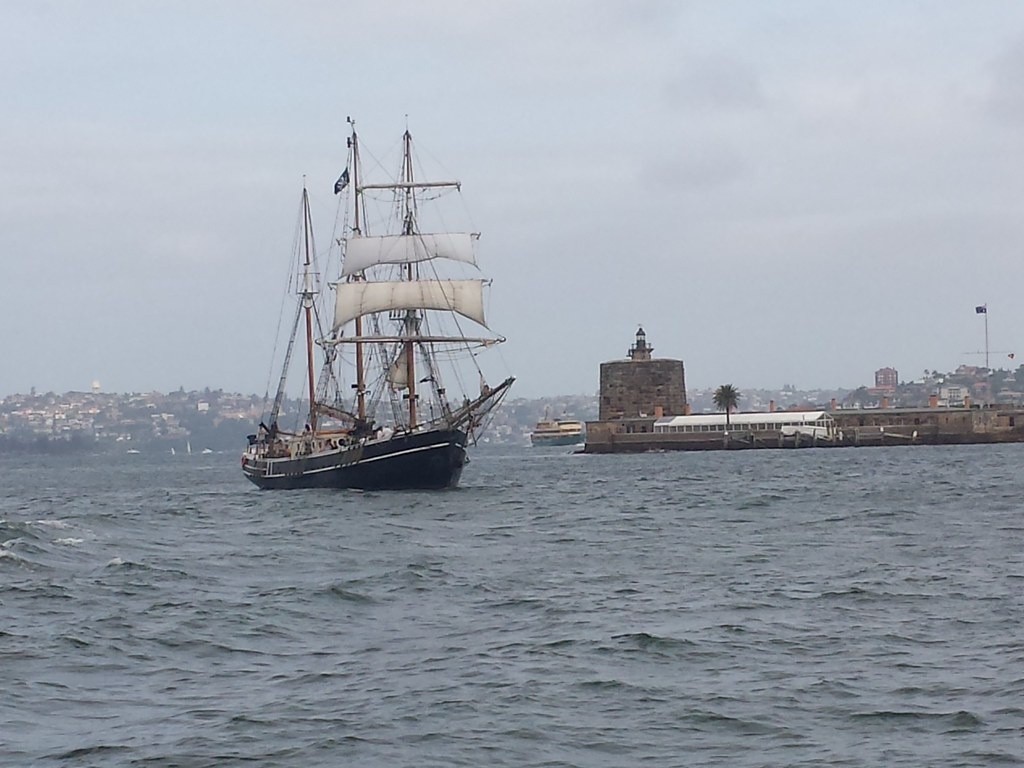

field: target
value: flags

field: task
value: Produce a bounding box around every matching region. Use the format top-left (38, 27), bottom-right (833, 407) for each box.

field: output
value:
top-left (334, 167), bottom-right (350, 196)
top-left (976, 307), bottom-right (986, 314)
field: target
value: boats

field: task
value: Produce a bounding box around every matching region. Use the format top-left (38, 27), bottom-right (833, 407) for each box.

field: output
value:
top-left (530, 418), bottom-right (585, 447)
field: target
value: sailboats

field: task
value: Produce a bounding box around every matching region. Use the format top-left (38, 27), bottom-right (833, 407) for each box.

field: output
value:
top-left (240, 117), bottom-right (517, 495)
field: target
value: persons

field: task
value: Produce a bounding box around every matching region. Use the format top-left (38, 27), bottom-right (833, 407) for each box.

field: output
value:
top-left (319, 438), bottom-right (338, 452)
top-left (275, 439), bottom-right (291, 458)
top-left (377, 425), bottom-right (391, 441)
